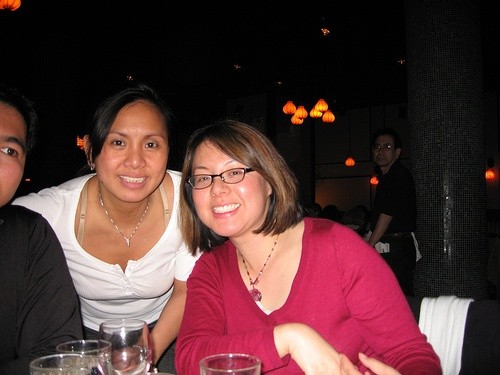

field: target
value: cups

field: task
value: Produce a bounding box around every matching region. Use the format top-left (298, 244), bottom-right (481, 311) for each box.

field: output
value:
top-left (97, 318), bottom-right (152, 375)
top-left (30, 353), bottom-right (92, 375)
top-left (199, 352), bottom-right (261, 375)
top-left (56, 340), bottom-right (113, 375)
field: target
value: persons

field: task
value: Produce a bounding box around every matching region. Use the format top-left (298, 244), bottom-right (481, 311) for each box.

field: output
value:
top-left (174, 120), bottom-right (443, 375)
top-left (364, 127), bottom-right (418, 296)
top-left (0, 87), bottom-right (87, 375)
top-left (302, 202), bottom-right (342, 221)
top-left (10, 85), bottom-right (212, 375)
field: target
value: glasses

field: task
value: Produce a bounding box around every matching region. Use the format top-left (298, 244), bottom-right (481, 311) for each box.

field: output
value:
top-left (182, 167), bottom-right (258, 190)
top-left (371, 144), bottom-right (394, 153)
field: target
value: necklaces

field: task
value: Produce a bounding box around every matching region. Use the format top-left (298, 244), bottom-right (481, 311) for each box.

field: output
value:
top-left (98, 181), bottom-right (150, 247)
top-left (241, 234), bottom-right (280, 301)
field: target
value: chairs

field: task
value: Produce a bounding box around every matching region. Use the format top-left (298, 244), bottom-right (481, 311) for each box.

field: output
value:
top-left (403, 296), bottom-right (500, 375)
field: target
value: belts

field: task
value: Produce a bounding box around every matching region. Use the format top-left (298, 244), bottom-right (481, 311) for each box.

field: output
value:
top-left (383, 233), bottom-right (412, 237)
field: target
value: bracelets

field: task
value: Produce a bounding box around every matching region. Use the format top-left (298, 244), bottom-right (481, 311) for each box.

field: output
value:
top-left (133, 345), bottom-right (147, 361)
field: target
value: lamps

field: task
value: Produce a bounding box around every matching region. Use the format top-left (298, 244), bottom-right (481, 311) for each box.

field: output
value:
top-left (282, 98), bottom-right (336, 126)
